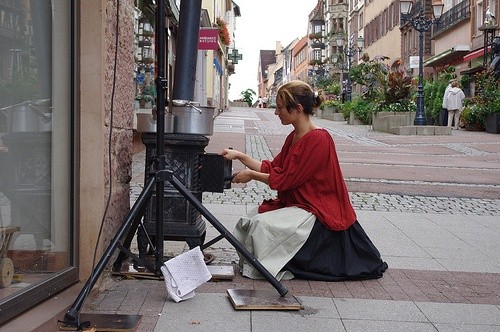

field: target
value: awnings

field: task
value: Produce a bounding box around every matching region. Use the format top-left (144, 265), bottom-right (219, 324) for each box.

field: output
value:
top-left (422, 45), bottom-right (470, 68)
top-left (462, 45), bottom-right (494, 63)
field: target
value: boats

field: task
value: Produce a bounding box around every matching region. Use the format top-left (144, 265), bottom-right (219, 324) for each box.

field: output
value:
top-left (336, 36), bottom-right (365, 102)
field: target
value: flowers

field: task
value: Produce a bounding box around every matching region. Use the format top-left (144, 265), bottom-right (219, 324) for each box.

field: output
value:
top-left (217, 19), bottom-right (230, 45)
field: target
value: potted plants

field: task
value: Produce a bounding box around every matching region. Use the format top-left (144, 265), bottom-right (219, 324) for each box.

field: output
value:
top-left (134, 95), bottom-right (151, 108)
top-left (463, 106), bottom-right (485, 131)
top-left (480, 104), bottom-right (500, 133)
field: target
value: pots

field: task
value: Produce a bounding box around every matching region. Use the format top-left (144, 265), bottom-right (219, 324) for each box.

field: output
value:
top-left (165, 101), bottom-right (222, 135)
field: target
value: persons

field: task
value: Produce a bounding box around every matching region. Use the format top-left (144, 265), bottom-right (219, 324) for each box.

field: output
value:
top-left (258, 96), bottom-right (265, 108)
top-left (439, 78), bottom-right (457, 126)
top-left (446, 81), bottom-right (465, 130)
top-left (218, 80), bottom-right (389, 281)
top-left (262, 95), bottom-right (268, 108)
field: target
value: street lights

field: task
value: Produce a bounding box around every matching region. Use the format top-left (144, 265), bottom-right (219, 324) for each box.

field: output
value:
top-left (400, 0), bottom-right (444, 126)
